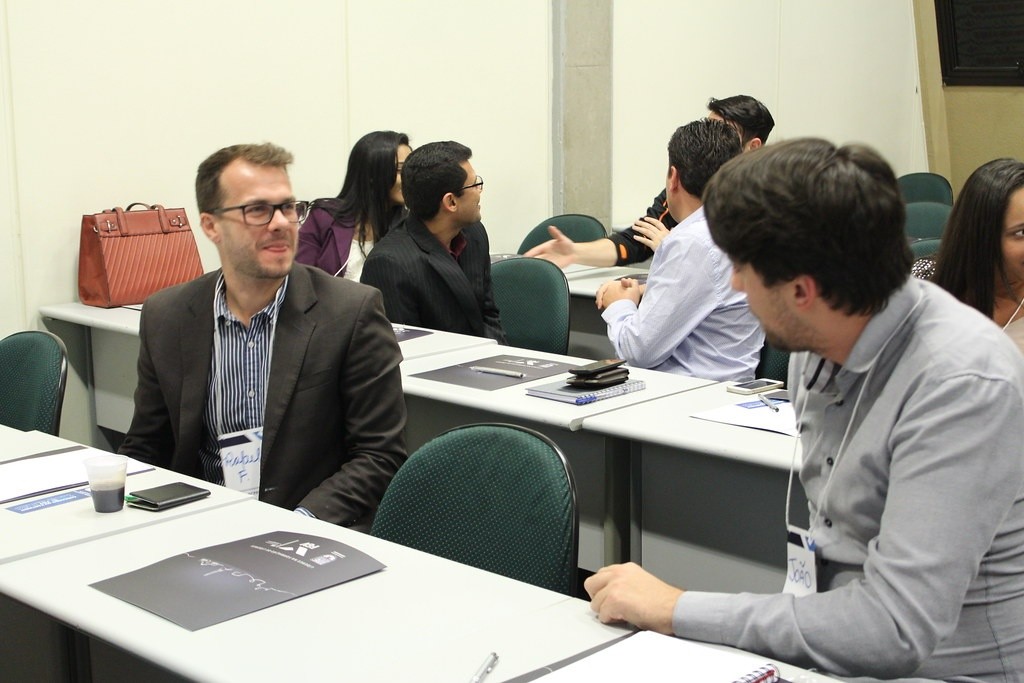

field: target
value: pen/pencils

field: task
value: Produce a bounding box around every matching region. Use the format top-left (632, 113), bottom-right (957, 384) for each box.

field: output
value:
top-left (468, 365), bottom-right (523, 378)
top-left (470, 652), bottom-right (499, 682)
top-left (758, 393), bottom-right (779, 412)
top-left (124, 495), bottom-right (141, 503)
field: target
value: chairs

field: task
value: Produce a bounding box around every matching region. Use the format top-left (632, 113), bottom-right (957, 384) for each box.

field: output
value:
top-left (490, 257), bottom-right (569, 354)
top-left (897, 169), bottom-right (958, 268)
top-left (371, 425), bottom-right (574, 604)
top-left (518, 213), bottom-right (606, 260)
top-left (0, 331), bottom-right (70, 437)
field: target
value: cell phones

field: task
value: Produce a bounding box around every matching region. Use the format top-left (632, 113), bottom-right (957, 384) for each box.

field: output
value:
top-left (569, 359), bottom-right (628, 373)
top-left (727, 378), bottom-right (784, 395)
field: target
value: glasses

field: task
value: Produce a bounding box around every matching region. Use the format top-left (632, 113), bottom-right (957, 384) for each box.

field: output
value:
top-left (205, 200), bottom-right (311, 227)
top-left (440, 174), bottom-right (484, 204)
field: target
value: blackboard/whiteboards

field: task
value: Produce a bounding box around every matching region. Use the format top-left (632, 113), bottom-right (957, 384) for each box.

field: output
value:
top-left (933, 0), bottom-right (1024, 86)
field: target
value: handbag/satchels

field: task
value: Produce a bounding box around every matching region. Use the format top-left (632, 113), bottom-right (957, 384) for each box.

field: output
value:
top-left (78, 202), bottom-right (206, 310)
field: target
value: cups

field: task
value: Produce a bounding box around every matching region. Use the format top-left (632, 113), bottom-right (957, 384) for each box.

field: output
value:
top-left (81, 454), bottom-right (129, 513)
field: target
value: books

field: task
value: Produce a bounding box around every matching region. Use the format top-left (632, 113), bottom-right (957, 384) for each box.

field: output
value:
top-left (526, 379), bottom-right (646, 405)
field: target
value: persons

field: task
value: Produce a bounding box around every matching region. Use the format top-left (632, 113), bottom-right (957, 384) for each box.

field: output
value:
top-left (523, 96), bottom-right (775, 267)
top-left (360, 140), bottom-right (507, 345)
top-left (296, 131), bottom-right (413, 284)
top-left (584, 137), bottom-right (1024, 682)
top-left (912, 159), bottom-right (1024, 356)
top-left (595, 119), bottom-right (768, 382)
top-left (117, 142), bottom-right (408, 532)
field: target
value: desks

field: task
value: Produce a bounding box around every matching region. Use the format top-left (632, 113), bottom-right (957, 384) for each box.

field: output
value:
top-left (0, 259), bottom-right (953, 683)
